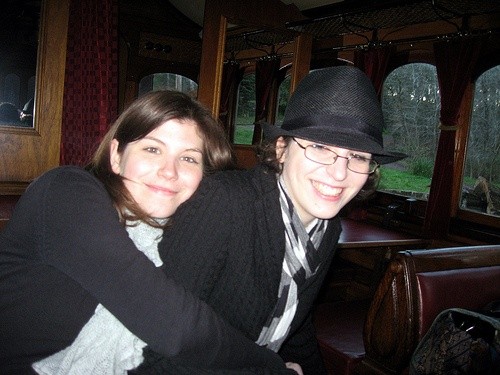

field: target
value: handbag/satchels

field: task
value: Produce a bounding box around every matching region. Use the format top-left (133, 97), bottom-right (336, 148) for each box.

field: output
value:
top-left (408, 307), bottom-right (500, 375)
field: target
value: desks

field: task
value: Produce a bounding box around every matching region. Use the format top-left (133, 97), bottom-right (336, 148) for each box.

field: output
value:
top-left (335, 217), bottom-right (426, 250)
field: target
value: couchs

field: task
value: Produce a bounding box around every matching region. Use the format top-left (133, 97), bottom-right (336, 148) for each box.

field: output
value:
top-left (313, 245), bottom-right (500, 374)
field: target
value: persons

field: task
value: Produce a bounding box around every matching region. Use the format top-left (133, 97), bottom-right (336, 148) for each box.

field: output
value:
top-left (20, 98), bottom-right (35, 127)
top-left (0, 90), bottom-right (304, 375)
top-left (128, 66), bottom-right (410, 375)
top-left (0, 102), bottom-right (20, 125)
top-left (465, 176), bottom-right (490, 214)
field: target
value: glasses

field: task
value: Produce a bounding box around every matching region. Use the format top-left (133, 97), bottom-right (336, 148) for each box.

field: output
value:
top-left (289, 136), bottom-right (380, 174)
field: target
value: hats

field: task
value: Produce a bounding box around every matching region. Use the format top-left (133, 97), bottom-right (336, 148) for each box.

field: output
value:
top-left (259, 66), bottom-right (409, 165)
